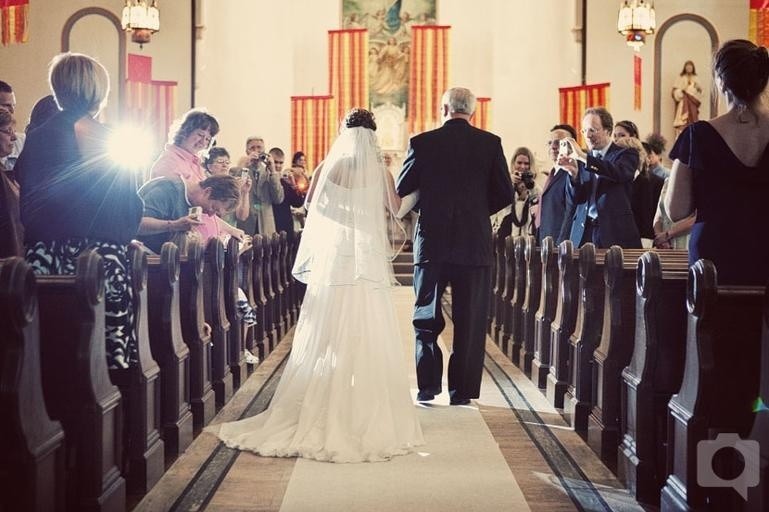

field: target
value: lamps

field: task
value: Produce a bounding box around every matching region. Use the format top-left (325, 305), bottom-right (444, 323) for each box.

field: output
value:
top-left (607, 0), bottom-right (661, 52)
top-left (113, 0), bottom-right (167, 48)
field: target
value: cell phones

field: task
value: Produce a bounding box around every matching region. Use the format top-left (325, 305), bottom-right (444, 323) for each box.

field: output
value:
top-left (238, 167), bottom-right (250, 181)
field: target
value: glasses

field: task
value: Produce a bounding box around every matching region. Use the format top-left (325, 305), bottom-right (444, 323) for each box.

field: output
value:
top-left (208, 157), bottom-right (230, 165)
top-left (0, 127), bottom-right (16, 135)
top-left (580, 127), bottom-right (603, 134)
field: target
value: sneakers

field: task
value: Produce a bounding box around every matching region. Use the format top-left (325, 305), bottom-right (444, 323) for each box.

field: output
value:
top-left (245, 350), bottom-right (258, 364)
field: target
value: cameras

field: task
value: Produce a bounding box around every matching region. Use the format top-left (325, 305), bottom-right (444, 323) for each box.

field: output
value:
top-left (257, 152), bottom-right (270, 164)
top-left (519, 171), bottom-right (536, 191)
top-left (558, 137), bottom-right (569, 156)
top-left (278, 171), bottom-right (291, 179)
top-left (188, 204), bottom-right (203, 220)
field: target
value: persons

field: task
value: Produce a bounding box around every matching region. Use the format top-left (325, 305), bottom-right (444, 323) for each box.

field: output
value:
top-left (393, 84), bottom-right (517, 408)
top-left (12, 49), bottom-right (148, 370)
top-left (344, 7), bottom-right (438, 109)
top-left (303, 109), bottom-right (415, 410)
top-left (670, 58), bottom-right (706, 143)
top-left (661, 37), bottom-right (769, 286)
top-left (2, 82), bottom-right (714, 366)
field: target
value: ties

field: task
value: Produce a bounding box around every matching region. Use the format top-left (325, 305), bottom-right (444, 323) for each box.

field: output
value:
top-left (588, 153), bottom-right (598, 219)
top-left (535, 168), bottom-right (555, 228)
